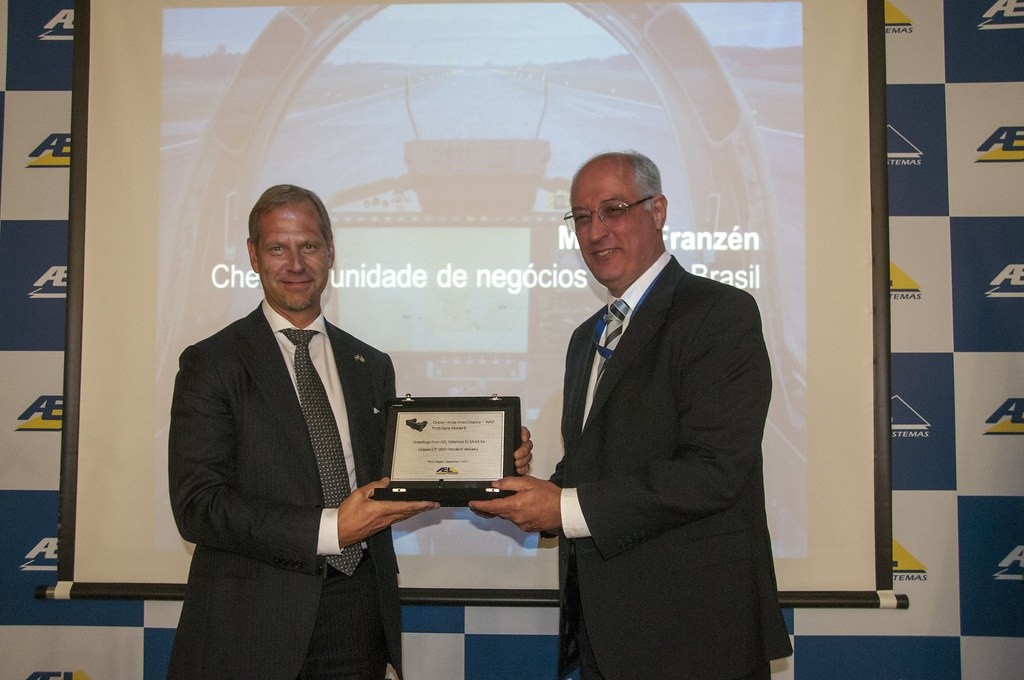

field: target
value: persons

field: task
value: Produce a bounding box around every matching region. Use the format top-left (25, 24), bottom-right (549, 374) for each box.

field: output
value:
top-left (168, 186), bottom-right (533, 680)
top-left (470, 150), bottom-right (792, 680)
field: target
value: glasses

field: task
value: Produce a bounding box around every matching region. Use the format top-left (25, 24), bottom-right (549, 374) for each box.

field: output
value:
top-left (564, 196), bottom-right (656, 232)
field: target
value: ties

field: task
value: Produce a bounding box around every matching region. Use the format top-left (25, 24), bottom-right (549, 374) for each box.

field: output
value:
top-left (281, 327), bottom-right (364, 575)
top-left (593, 300), bottom-right (630, 399)
